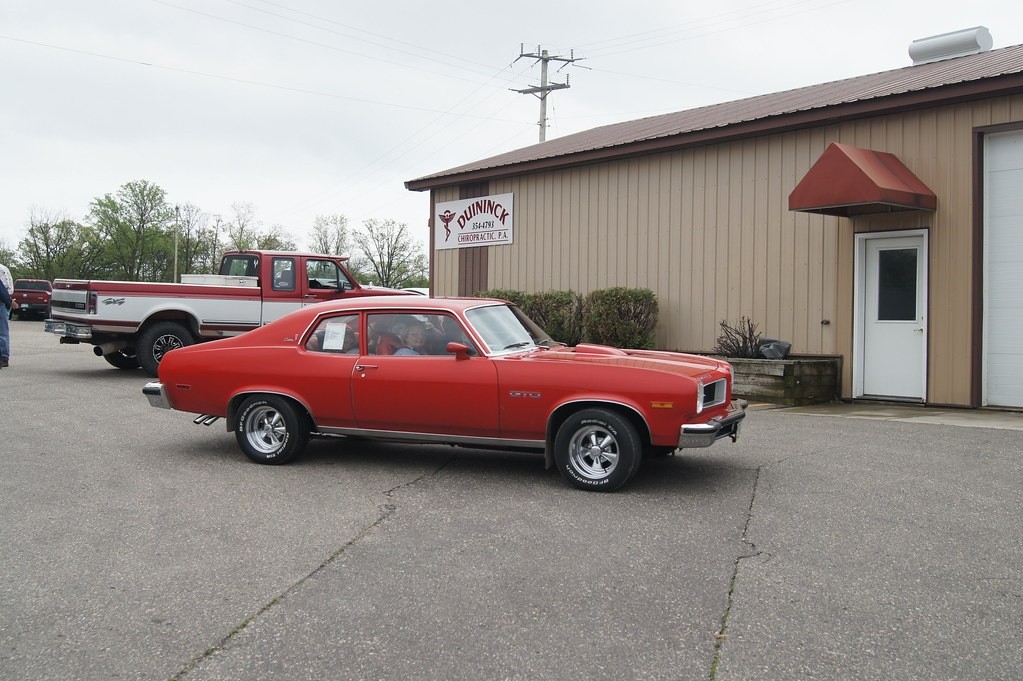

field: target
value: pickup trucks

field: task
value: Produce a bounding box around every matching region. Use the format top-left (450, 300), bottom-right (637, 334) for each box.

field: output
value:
top-left (44, 249), bottom-right (418, 377)
top-left (9, 279), bottom-right (53, 320)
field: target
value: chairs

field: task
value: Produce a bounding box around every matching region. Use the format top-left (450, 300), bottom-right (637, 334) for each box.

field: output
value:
top-left (374, 332), bottom-right (402, 356)
top-left (315, 330), bottom-right (360, 354)
top-left (281, 269), bottom-right (293, 282)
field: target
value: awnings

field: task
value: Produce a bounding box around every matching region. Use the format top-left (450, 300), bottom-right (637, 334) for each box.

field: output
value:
top-left (788, 142), bottom-right (937, 217)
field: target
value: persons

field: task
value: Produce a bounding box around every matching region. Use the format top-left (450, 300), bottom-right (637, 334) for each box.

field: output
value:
top-left (433, 316), bottom-right (475, 354)
top-left (0, 264), bottom-right (18, 368)
top-left (340, 323), bottom-right (386, 355)
top-left (394, 322), bottom-right (432, 355)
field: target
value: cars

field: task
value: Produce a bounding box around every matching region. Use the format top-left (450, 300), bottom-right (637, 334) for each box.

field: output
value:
top-left (141, 294), bottom-right (747, 493)
